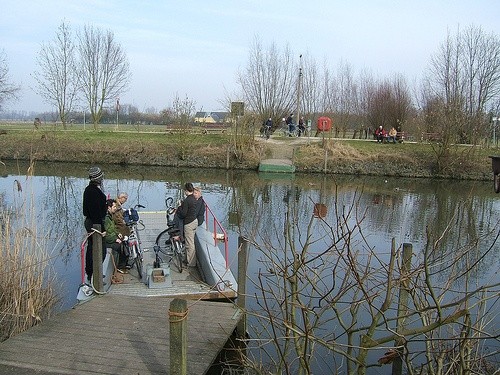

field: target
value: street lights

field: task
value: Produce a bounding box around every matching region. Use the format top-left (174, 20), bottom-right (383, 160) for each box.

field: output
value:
top-left (492, 116), bottom-right (497, 145)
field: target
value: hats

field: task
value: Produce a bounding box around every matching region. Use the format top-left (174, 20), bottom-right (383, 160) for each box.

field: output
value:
top-left (89, 167), bottom-right (104, 184)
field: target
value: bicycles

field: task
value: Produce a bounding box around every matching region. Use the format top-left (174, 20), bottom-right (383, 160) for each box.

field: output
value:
top-left (155, 197), bottom-right (184, 273)
top-left (260, 124), bottom-right (272, 140)
top-left (113, 204), bottom-right (150, 279)
top-left (291, 124), bottom-right (310, 138)
top-left (280, 124), bottom-right (302, 137)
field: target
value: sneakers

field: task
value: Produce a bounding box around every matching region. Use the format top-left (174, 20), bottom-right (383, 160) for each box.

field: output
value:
top-left (117, 264), bottom-right (132, 274)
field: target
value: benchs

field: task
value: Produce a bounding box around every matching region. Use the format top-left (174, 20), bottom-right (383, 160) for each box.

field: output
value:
top-left (383, 131), bottom-right (407, 144)
top-left (421, 132), bottom-right (444, 142)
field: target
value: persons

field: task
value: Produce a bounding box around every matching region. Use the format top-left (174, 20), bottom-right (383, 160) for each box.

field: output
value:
top-left (298, 116), bottom-right (306, 137)
top-left (387, 126), bottom-right (398, 144)
top-left (82, 167), bottom-right (109, 286)
top-left (264, 117), bottom-right (273, 140)
top-left (113, 191), bottom-right (129, 228)
top-left (286, 113), bottom-right (296, 137)
top-left (103, 198), bottom-right (133, 274)
top-left (164, 187), bottom-right (205, 244)
top-left (176, 182), bottom-right (199, 268)
top-left (375, 125), bottom-right (387, 143)
